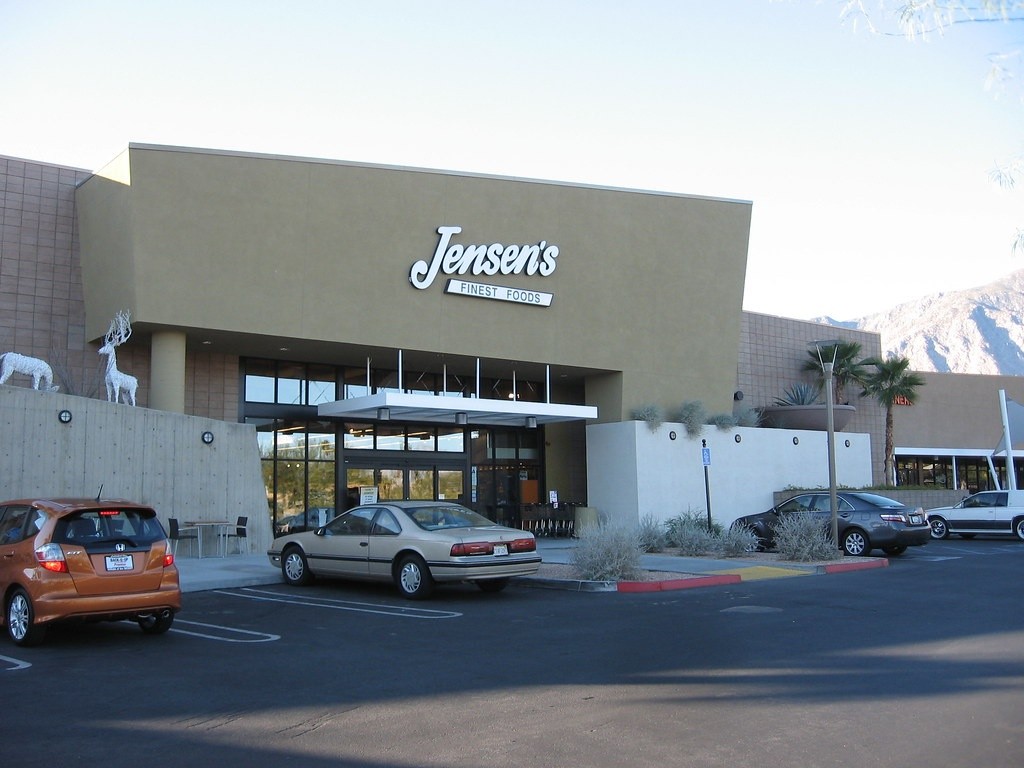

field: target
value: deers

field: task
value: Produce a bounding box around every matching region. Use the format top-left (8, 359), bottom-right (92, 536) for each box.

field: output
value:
top-left (97, 307), bottom-right (140, 408)
top-left (0, 352), bottom-right (60, 392)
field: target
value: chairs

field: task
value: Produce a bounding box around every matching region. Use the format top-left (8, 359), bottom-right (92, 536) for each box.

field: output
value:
top-left (216, 516), bottom-right (249, 557)
top-left (167, 518), bottom-right (199, 561)
top-left (71, 518), bottom-right (97, 540)
top-left (818, 498), bottom-right (826, 511)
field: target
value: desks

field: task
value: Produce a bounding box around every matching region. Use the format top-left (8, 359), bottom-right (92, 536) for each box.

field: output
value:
top-left (183, 520), bottom-right (230, 559)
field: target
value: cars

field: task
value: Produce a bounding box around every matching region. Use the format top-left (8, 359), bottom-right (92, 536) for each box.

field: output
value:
top-left (275, 514), bottom-right (294, 536)
top-left (926, 489), bottom-right (1024, 540)
top-left (728, 491), bottom-right (932, 556)
top-left (288, 508), bottom-right (337, 535)
top-left (266, 499), bottom-right (543, 600)
top-left (0, 494), bottom-right (184, 645)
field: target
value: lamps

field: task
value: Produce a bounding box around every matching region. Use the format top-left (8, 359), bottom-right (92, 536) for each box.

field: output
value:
top-left (525, 416), bottom-right (537, 428)
top-left (377, 408), bottom-right (390, 420)
top-left (455, 413), bottom-right (467, 424)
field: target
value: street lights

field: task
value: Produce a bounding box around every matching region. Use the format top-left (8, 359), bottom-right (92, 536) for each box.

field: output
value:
top-left (805, 338), bottom-right (847, 553)
